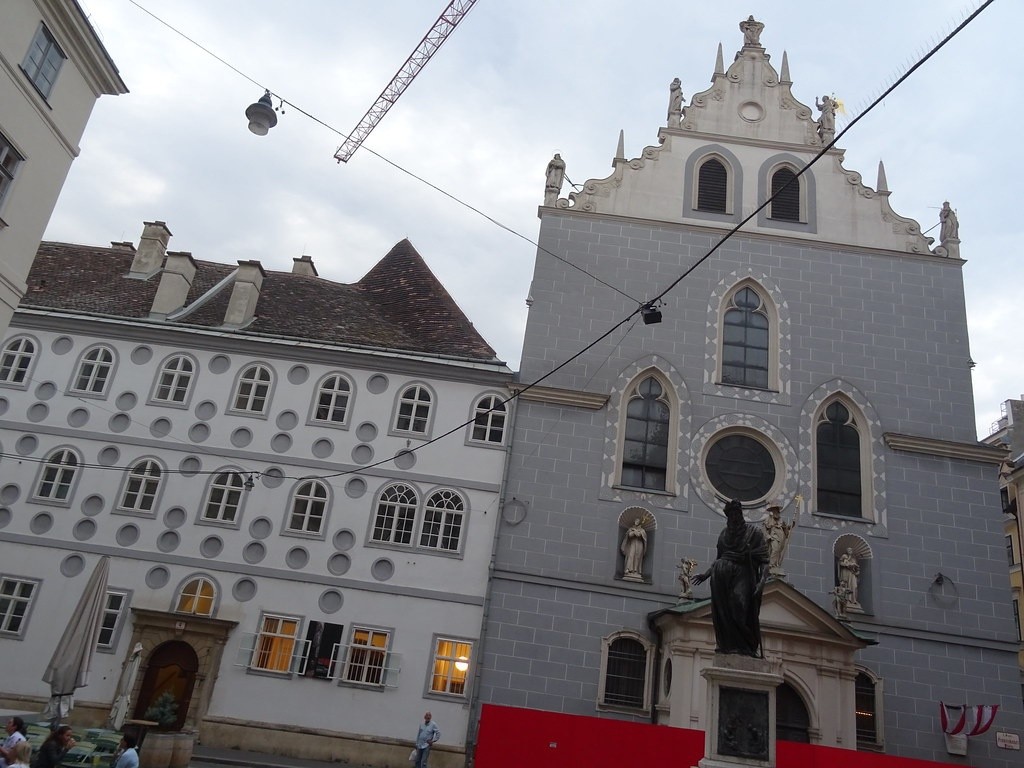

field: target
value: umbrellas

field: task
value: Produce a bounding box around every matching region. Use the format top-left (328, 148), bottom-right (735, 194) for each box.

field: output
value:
top-left (108, 641), bottom-right (144, 732)
top-left (39, 552), bottom-right (118, 734)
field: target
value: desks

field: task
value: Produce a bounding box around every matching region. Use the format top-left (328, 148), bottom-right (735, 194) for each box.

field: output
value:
top-left (61, 761), bottom-right (112, 768)
top-left (127, 719), bottom-right (159, 756)
top-left (0, 708), bottom-right (40, 724)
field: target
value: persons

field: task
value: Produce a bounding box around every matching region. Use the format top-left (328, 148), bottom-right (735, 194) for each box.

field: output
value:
top-left (826, 579), bottom-right (853, 617)
top-left (0, 714), bottom-right (29, 768)
top-left (763, 501), bottom-right (795, 575)
top-left (939, 199), bottom-right (961, 241)
top-left (813, 94), bottom-right (839, 136)
top-left (619, 516), bottom-right (651, 579)
top-left (545, 151), bottom-right (569, 192)
top-left (413, 711), bottom-right (443, 768)
top-left (111, 731), bottom-right (140, 768)
top-left (676, 555), bottom-right (698, 598)
top-left (666, 74), bottom-right (688, 117)
top-left (5, 739), bottom-right (32, 768)
top-left (690, 497), bottom-right (770, 660)
top-left (837, 545), bottom-right (866, 609)
top-left (37, 725), bottom-right (76, 768)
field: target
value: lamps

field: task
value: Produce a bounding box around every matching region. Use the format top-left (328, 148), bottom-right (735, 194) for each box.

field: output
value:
top-left (455, 643), bottom-right (470, 672)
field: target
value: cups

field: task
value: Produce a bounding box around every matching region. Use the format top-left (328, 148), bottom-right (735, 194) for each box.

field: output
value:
top-left (93, 755), bottom-right (101, 766)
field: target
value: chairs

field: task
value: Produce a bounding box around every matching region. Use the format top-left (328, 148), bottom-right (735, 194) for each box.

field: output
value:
top-left (0, 722), bottom-right (124, 768)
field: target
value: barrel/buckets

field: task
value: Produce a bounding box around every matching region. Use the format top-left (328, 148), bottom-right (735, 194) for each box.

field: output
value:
top-left (169, 732), bottom-right (194, 768)
top-left (139, 731), bottom-right (175, 768)
